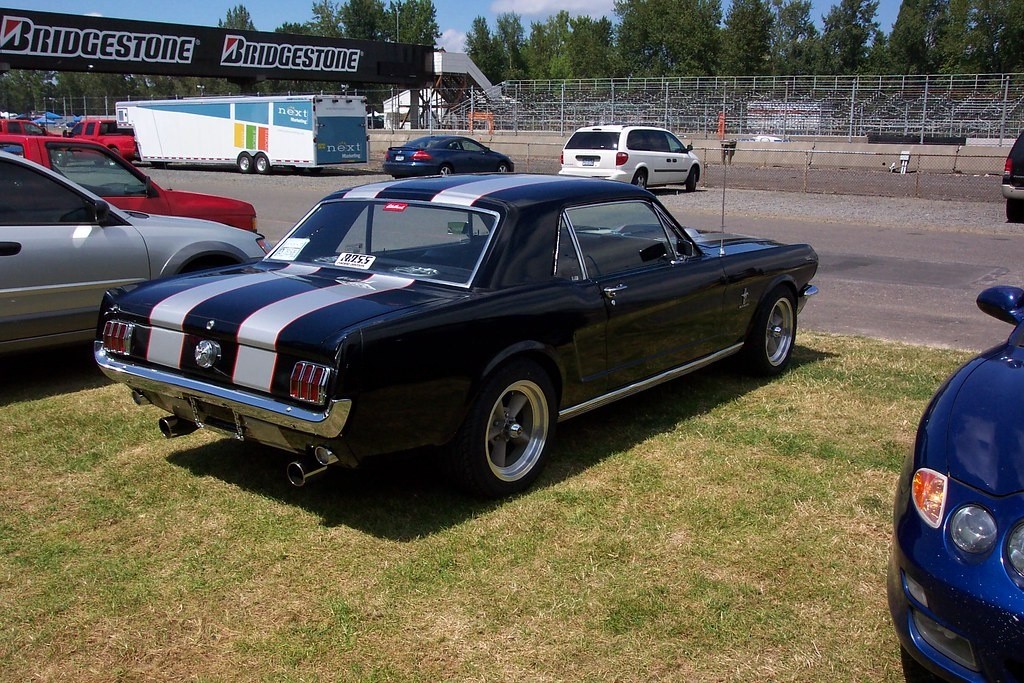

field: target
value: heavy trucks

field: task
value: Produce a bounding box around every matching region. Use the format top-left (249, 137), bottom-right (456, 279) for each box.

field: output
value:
top-left (115, 95), bottom-right (369, 175)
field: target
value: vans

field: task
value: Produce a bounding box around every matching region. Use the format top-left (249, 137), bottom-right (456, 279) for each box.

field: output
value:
top-left (558, 125), bottom-right (702, 189)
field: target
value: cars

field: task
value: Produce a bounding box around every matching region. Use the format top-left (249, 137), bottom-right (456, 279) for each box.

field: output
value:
top-left (1002, 129), bottom-right (1024, 223)
top-left (92, 181), bottom-right (819, 497)
top-left (381, 136), bottom-right (514, 179)
top-left (0, 134), bottom-right (257, 235)
top-left (0, 120), bottom-right (63, 137)
top-left (891, 286), bottom-right (1024, 683)
top-left (0, 150), bottom-right (271, 352)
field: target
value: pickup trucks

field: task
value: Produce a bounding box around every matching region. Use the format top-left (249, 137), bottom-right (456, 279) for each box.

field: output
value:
top-left (63, 119), bottom-right (140, 159)
